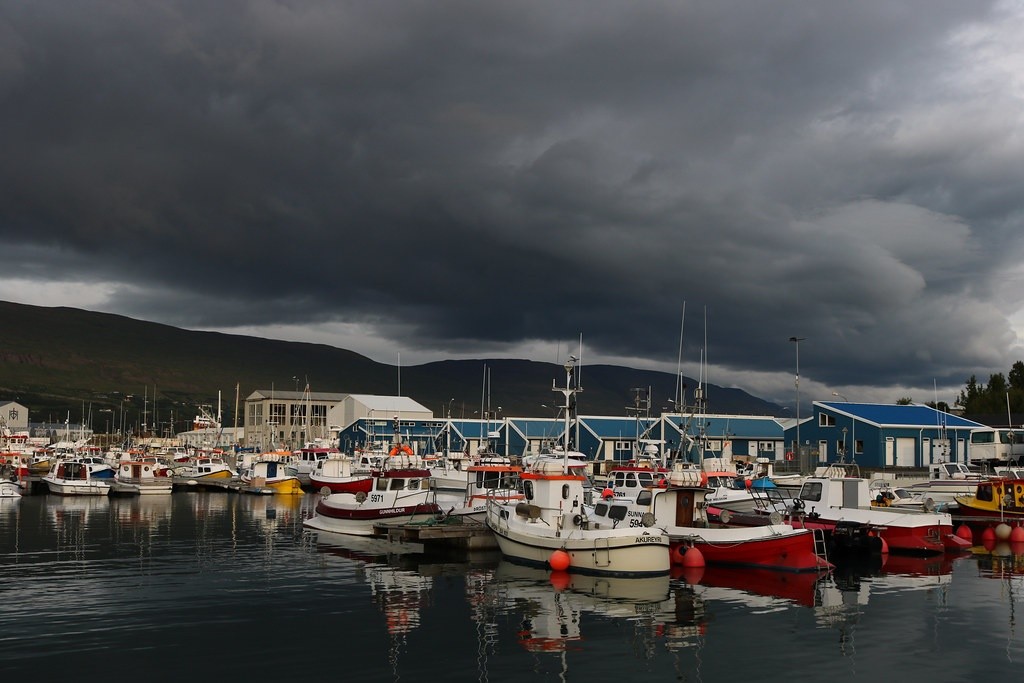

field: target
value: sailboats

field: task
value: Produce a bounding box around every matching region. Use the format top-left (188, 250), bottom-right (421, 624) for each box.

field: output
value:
top-left (302, 301), bottom-right (1024, 576)
top-left (0, 375), bottom-right (356, 497)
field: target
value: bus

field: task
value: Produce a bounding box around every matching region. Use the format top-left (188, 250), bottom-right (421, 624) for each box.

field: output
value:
top-left (970, 428), bottom-right (1024, 466)
